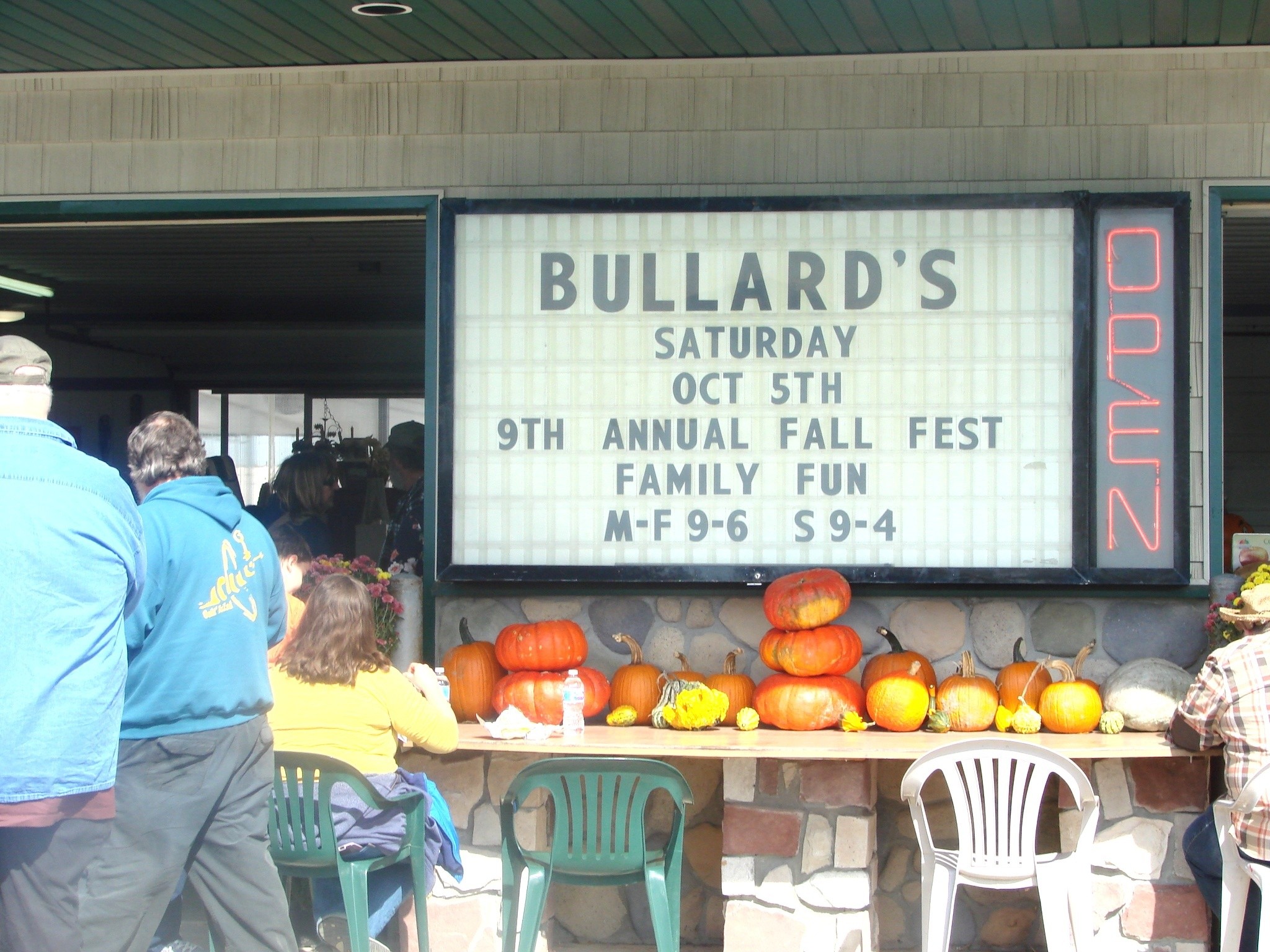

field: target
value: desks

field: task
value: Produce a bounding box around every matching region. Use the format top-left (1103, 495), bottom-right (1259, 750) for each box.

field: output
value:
top-left (455, 719), bottom-right (1225, 759)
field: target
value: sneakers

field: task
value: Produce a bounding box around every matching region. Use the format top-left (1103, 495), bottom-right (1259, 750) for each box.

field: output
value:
top-left (315, 913), bottom-right (391, 951)
top-left (166, 938), bottom-right (208, 952)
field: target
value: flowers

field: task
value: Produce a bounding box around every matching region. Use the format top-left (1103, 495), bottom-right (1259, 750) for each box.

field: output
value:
top-left (293, 554), bottom-right (419, 662)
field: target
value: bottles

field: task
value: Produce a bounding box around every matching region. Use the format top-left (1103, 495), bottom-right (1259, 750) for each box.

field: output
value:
top-left (434, 666), bottom-right (450, 704)
top-left (562, 669), bottom-right (585, 737)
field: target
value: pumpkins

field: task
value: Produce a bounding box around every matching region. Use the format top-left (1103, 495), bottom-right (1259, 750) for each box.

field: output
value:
top-left (443, 570), bottom-right (1194, 732)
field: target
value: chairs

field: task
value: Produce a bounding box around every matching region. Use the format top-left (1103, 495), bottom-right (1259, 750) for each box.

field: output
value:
top-left (898, 737), bottom-right (1102, 952)
top-left (268, 750), bottom-right (427, 952)
top-left (500, 757), bottom-right (693, 952)
top-left (1213, 762), bottom-right (1270, 952)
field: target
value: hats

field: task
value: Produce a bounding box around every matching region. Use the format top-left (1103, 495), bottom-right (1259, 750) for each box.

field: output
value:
top-left (0, 334), bottom-right (53, 387)
top-left (1218, 582), bottom-right (1270, 624)
top-left (382, 420), bottom-right (425, 454)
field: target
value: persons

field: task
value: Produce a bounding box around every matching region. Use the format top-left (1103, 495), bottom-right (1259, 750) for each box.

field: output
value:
top-left (75, 409), bottom-right (299, 952)
top-left (377, 420), bottom-right (425, 577)
top-left (265, 451), bottom-right (335, 560)
top-left (266, 573), bottom-right (459, 952)
top-left (1168, 583), bottom-right (1270, 952)
top-left (0, 333), bottom-right (149, 952)
top-left (266, 523), bottom-right (313, 671)
